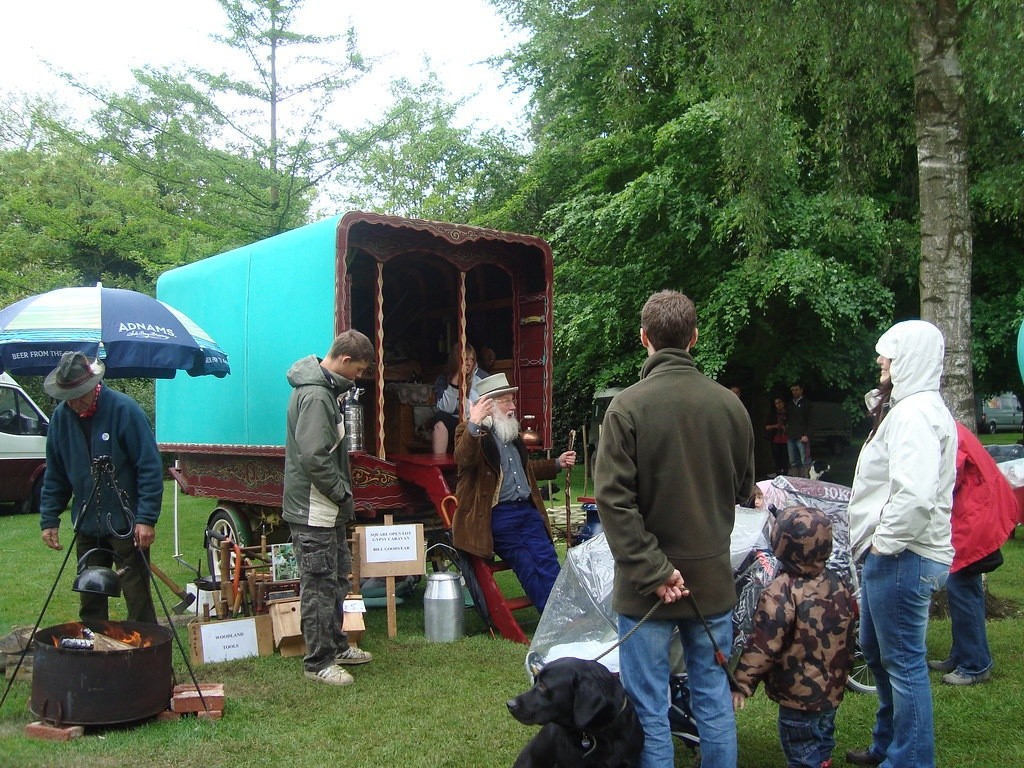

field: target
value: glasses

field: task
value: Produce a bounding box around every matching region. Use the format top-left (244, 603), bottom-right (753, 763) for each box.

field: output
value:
top-left (493, 398), bottom-right (518, 407)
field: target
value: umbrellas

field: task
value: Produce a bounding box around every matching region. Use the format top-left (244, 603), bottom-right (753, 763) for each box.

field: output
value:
top-left (0, 283), bottom-right (230, 379)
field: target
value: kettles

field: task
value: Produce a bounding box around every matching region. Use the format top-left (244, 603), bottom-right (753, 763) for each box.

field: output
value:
top-left (518, 415), bottom-right (541, 446)
top-left (71, 548), bottom-right (132, 598)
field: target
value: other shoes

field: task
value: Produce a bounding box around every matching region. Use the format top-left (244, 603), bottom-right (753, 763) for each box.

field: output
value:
top-left (789, 467), bottom-right (801, 477)
top-left (802, 467), bottom-right (811, 478)
top-left (846, 747), bottom-right (882, 766)
top-left (819, 750), bottom-right (832, 767)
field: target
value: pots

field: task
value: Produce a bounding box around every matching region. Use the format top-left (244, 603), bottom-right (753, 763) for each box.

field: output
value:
top-left (192, 575), bottom-right (241, 591)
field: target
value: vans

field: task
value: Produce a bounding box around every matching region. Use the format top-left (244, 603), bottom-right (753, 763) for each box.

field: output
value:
top-left (0, 370), bottom-right (51, 515)
top-left (976, 391), bottom-right (1024, 435)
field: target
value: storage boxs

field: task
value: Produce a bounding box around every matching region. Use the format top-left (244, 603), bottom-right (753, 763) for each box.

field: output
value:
top-left (188, 613), bottom-right (274, 668)
top-left (265, 596), bottom-right (306, 648)
top-left (340, 595), bottom-right (366, 643)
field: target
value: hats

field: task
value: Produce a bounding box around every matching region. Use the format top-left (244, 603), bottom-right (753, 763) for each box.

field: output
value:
top-left (474, 372), bottom-right (518, 405)
top-left (43, 350), bottom-right (106, 401)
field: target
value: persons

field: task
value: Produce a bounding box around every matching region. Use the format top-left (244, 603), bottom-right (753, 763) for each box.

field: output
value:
top-left (282, 330), bottom-right (373, 684)
top-left (433, 343), bottom-right (490, 455)
top-left (765, 396), bottom-right (789, 474)
top-left (40, 350), bottom-right (162, 625)
top-left (731, 384), bottom-right (742, 398)
top-left (785, 384), bottom-right (815, 477)
top-left (927, 423), bottom-right (1020, 685)
top-left (733, 507), bottom-right (855, 768)
top-left (594, 289), bottom-right (754, 768)
top-left (450, 373), bottom-right (576, 618)
top-left (846, 319), bottom-right (959, 768)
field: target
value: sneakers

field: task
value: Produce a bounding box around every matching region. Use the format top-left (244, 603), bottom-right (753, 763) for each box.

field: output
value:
top-left (304, 664), bottom-right (354, 686)
top-left (927, 657), bottom-right (958, 673)
top-left (941, 669), bottom-right (991, 685)
top-left (335, 646), bottom-right (372, 664)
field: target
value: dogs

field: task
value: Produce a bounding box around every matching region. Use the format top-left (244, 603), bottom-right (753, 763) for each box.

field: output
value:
top-left (505, 657), bottom-right (645, 768)
top-left (809, 461), bottom-right (830, 480)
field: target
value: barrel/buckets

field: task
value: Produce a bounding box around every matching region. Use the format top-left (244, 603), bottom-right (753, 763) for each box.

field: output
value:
top-left (422, 543), bottom-right (465, 644)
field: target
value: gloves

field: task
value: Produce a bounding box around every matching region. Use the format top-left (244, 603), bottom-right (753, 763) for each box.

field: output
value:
top-left (338, 496), bottom-right (357, 520)
